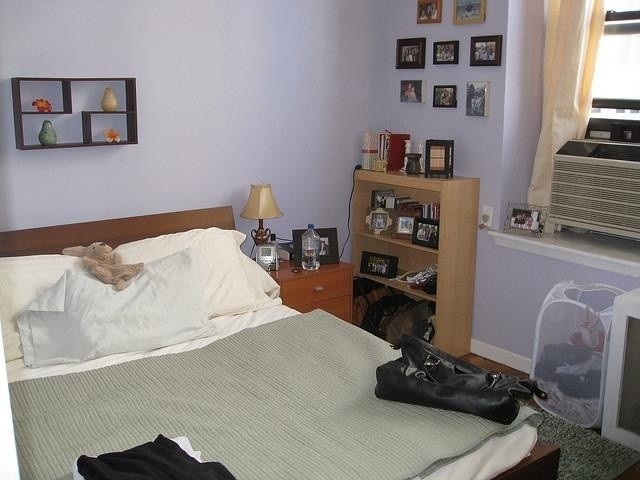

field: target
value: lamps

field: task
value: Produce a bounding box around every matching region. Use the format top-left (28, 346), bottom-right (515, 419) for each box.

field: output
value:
top-left (240, 182), bottom-right (283, 242)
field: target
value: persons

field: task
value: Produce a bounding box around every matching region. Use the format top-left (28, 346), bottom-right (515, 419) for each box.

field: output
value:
top-left (408, 87), bottom-right (417, 102)
top-left (404, 84), bottom-right (412, 96)
top-left (417, 223), bottom-right (437, 242)
top-left (404, 47), bottom-right (419, 63)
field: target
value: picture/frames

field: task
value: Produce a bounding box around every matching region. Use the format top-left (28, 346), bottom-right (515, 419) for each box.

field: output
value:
top-left (363, 205), bottom-right (394, 237)
top-left (360, 245), bottom-right (401, 278)
top-left (413, 219), bottom-right (440, 248)
top-left (293, 227), bottom-right (339, 265)
top-left (389, 209), bottom-right (419, 241)
top-left (503, 200), bottom-right (551, 238)
top-left (394, 0), bottom-right (505, 118)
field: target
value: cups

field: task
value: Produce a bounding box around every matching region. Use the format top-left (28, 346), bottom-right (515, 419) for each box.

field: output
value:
top-left (405, 153), bottom-right (422, 175)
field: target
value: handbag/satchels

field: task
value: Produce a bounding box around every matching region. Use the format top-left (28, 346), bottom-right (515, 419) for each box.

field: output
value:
top-left (361, 294), bottom-right (416, 339)
top-left (397, 264), bottom-right (437, 284)
top-left (382, 298), bottom-right (436, 345)
top-left (353, 286), bottom-right (394, 326)
top-left (410, 274), bottom-right (437, 295)
top-left (374, 332), bottom-right (548, 425)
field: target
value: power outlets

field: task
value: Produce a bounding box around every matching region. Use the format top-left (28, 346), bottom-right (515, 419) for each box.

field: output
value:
top-left (480, 205), bottom-right (494, 230)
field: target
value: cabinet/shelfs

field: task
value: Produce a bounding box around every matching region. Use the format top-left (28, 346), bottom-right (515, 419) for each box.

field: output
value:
top-left (599, 286), bottom-right (640, 451)
top-left (349, 168), bottom-right (482, 357)
top-left (11, 75), bottom-right (140, 150)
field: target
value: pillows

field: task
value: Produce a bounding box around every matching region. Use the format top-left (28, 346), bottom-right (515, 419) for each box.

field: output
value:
top-left (0, 226), bottom-right (281, 368)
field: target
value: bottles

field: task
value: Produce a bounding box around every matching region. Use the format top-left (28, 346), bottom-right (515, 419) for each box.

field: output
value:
top-left (301, 223), bottom-right (321, 271)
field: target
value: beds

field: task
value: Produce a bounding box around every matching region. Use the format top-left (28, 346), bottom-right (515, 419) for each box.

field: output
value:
top-left (1, 205), bottom-right (561, 480)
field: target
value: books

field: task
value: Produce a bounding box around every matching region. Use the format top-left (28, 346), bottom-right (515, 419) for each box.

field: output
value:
top-left (362, 130), bottom-right (410, 170)
top-left (371, 190), bottom-right (439, 221)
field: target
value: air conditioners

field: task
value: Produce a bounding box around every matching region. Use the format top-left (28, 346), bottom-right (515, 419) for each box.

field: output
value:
top-left (542, 136), bottom-right (640, 245)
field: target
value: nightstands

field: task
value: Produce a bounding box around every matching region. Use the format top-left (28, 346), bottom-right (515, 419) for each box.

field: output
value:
top-left (249, 254), bottom-right (354, 324)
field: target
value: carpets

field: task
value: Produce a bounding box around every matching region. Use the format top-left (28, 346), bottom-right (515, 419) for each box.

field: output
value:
top-left (530, 399), bottom-right (639, 479)
